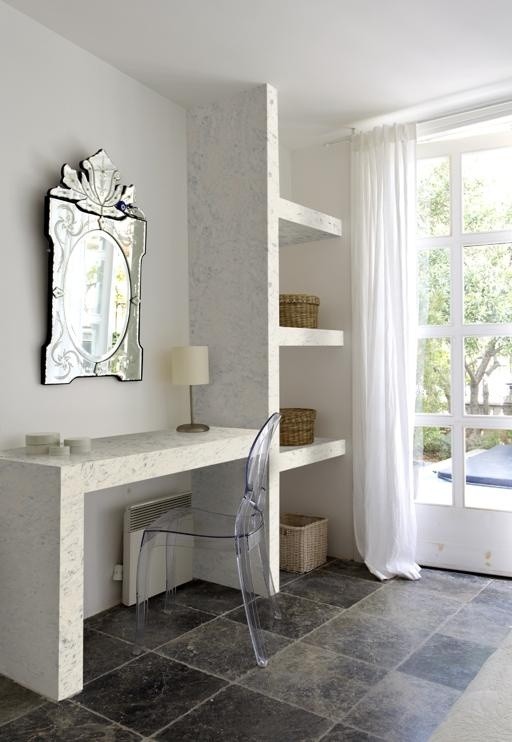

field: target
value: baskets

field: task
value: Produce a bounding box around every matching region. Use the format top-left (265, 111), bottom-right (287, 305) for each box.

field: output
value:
top-left (278, 406), bottom-right (319, 447)
top-left (277, 292), bottom-right (324, 329)
top-left (275, 511), bottom-right (332, 575)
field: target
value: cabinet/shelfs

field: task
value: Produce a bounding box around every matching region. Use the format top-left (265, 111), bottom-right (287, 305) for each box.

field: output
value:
top-left (274, 193), bottom-right (350, 475)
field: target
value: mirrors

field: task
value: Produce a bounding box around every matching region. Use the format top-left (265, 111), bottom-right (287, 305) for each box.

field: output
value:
top-left (31, 145), bottom-right (149, 387)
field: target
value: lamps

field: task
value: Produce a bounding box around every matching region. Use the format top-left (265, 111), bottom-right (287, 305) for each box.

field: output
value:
top-left (166, 344), bottom-right (215, 435)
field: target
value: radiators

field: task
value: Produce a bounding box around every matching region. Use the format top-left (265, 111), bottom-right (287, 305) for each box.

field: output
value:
top-left (122, 490), bottom-right (194, 610)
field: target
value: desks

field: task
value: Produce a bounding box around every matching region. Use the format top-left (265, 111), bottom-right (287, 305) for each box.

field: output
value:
top-left (0, 425), bottom-right (261, 702)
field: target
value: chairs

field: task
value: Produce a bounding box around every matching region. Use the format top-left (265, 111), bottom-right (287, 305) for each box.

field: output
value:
top-left (125, 405), bottom-right (292, 669)
